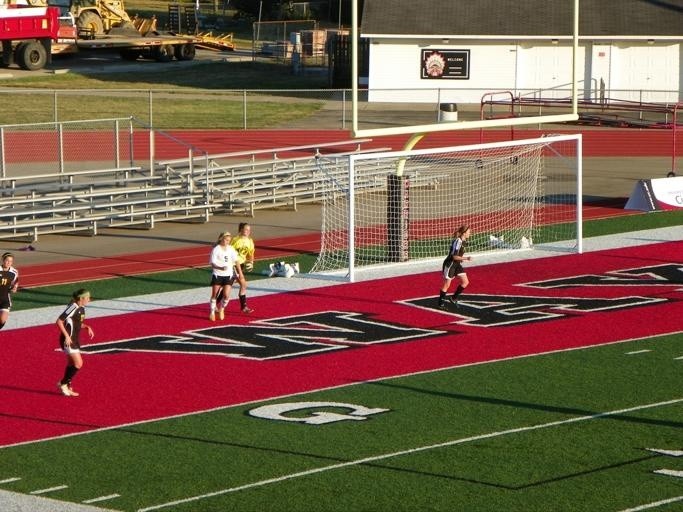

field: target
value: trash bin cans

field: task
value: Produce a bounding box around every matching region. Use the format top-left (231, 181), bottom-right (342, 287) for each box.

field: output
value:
top-left (440, 103), bottom-right (458, 122)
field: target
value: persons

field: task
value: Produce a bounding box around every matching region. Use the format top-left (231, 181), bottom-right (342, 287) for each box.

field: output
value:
top-left (55, 289), bottom-right (94, 396)
top-left (438, 226), bottom-right (474, 311)
top-left (0, 252), bottom-right (18, 330)
top-left (215, 222), bottom-right (256, 313)
top-left (207, 232), bottom-right (244, 321)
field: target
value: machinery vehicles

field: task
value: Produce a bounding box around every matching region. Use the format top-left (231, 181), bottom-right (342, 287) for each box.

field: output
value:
top-left (68, 0), bottom-right (157, 36)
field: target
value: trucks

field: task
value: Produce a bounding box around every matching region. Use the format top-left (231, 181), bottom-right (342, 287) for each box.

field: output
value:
top-left (0, 1), bottom-right (234, 70)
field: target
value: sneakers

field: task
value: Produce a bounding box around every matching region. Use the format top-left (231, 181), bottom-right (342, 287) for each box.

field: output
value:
top-left (209, 305), bottom-right (255, 322)
top-left (57, 381), bottom-right (78, 396)
top-left (438, 297), bottom-right (460, 309)
